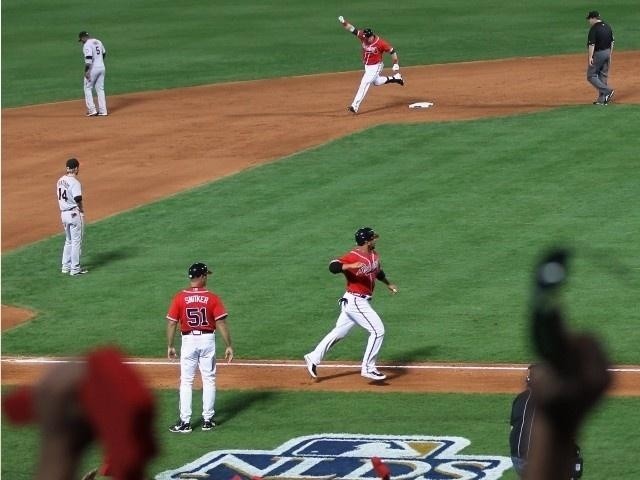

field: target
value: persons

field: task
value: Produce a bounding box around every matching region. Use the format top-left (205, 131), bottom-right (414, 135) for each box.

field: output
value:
top-left (509, 363), bottom-right (587, 479)
top-left (76, 30), bottom-right (110, 119)
top-left (302, 225), bottom-right (399, 382)
top-left (165, 262), bottom-right (237, 434)
top-left (56, 157), bottom-right (90, 277)
top-left (505, 245), bottom-right (620, 479)
top-left (338, 13), bottom-right (405, 113)
top-left (583, 9), bottom-right (616, 106)
top-left (2, 341), bottom-right (168, 480)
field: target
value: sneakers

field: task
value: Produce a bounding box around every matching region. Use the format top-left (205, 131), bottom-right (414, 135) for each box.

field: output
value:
top-left (361, 370), bottom-right (386, 380)
top-left (593, 89), bottom-right (615, 105)
top-left (169, 422), bottom-right (192, 432)
top-left (86, 112), bottom-right (108, 116)
top-left (202, 419), bottom-right (216, 431)
top-left (305, 355), bottom-right (318, 378)
top-left (394, 73), bottom-right (403, 86)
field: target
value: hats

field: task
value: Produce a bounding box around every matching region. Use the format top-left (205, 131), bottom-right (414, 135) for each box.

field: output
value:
top-left (79, 31), bottom-right (88, 42)
top-left (586, 11), bottom-right (599, 18)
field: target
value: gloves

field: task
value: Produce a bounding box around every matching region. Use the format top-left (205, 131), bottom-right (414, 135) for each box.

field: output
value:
top-left (338, 16), bottom-right (345, 24)
top-left (392, 63), bottom-right (399, 72)
top-left (338, 298), bottom-right (348, 306)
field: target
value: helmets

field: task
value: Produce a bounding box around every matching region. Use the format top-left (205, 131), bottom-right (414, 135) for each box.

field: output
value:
top-left (355, 228), bottom-right (380, 244)
top-left (362, 29), bottom-right (373, 35)
top-left (188, 262), bottom-right (212, 279)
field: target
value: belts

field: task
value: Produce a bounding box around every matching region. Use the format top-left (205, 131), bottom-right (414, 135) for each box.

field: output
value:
top-left (183, 329), bottom-right (214, 335)
top-left (348, 291), bottom-right (372, 301)
